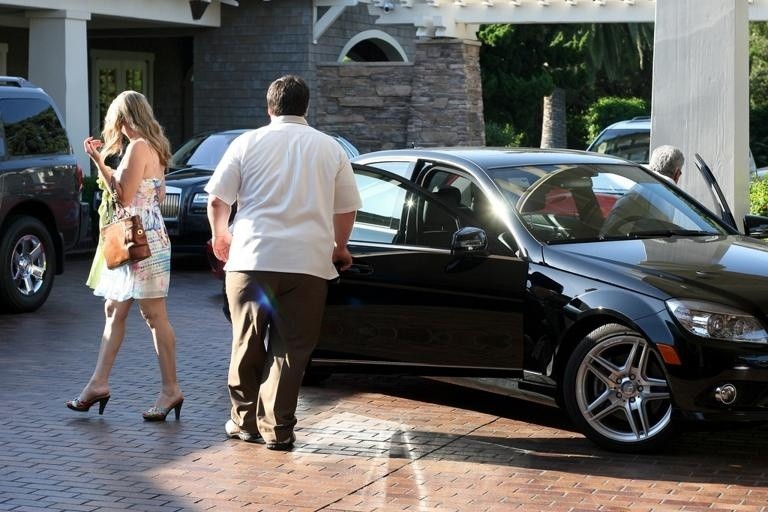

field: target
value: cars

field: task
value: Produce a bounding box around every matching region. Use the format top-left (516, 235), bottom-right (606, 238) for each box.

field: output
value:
top-left (90, 128), bottom-right (362, 255)
top-left (223, 146), bottom-right (768, 455)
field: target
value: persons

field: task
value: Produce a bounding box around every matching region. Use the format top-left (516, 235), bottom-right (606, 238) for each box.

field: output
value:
top-left (62, 88), bottom-right (187, 421)
top-left (202, 71), bottom-right (365, 452)
top-left (598, 143), bottom-right (686, 240)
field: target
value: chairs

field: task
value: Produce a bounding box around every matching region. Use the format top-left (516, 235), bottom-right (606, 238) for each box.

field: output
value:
top-left (468, 181), bottom-right (520, 256)
top-left (416, 187), bottom-right (474, 249)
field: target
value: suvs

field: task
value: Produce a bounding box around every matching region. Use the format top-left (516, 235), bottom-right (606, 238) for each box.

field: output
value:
top-left (0, 76), bottom-right (84, 315)
top-left (585, 114), bottom-right (758, 182)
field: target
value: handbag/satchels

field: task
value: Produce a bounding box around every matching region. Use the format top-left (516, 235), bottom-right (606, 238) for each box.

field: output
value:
top-left (101, 215), bottom-right (151, 270)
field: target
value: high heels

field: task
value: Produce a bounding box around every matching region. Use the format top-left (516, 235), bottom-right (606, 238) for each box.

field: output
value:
top-left (67, 393), bottom-right (110, 415)
top-left (143, 398), bottom-right (184, 421)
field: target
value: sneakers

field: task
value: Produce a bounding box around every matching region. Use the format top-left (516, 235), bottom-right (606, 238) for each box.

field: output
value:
top-left (266, 432), bottom-right (296, 451)
top-left (224, 419), bottom-right (262, 442)
